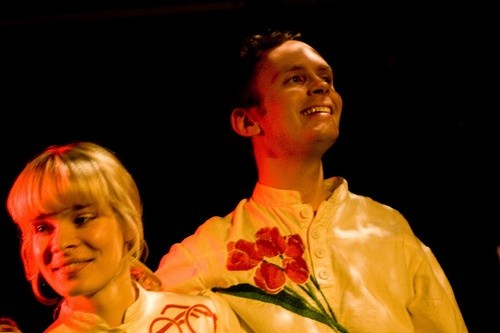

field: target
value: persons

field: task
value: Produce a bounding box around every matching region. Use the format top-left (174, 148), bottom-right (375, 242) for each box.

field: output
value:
top-left (153, 31), bottom-right (469, 333)
top-left (6, 143), bottom-right (255, 333)
